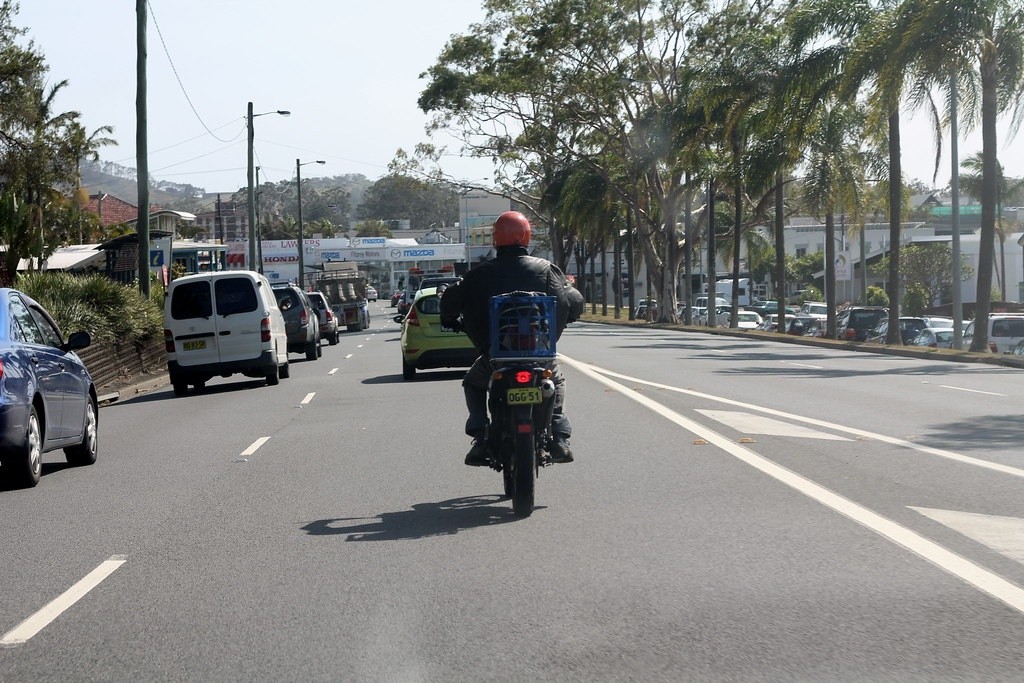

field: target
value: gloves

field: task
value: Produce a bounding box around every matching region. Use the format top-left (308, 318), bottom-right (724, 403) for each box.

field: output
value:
top-left (451, 317), bottom-right (462, 334)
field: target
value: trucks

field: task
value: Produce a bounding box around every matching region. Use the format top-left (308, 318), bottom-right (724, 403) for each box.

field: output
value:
top-left (308, 269), bottom-right (371, 332)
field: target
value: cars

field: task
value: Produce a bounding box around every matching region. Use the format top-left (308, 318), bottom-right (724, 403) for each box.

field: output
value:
top-left (0, 286), bottom-right (98, 486)
top-left (368, 286), bottom-right (378, 302)
top-left (634, 277), bottom-right (1024, 355)
top-left (389, 266), bottom-right (478, 381)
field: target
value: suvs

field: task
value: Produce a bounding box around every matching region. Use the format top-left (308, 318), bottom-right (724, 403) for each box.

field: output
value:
top-left (270, 281), bottom-right (323, 361)
top-left (306, 292), bottom-right (340, 347)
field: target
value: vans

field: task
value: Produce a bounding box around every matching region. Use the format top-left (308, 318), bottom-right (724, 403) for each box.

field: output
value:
top-left (163, 269), bottom-right (290, 395)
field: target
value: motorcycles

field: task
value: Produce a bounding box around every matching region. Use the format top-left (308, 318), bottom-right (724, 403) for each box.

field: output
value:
top-left (479, 291), bottom-right (556, 517)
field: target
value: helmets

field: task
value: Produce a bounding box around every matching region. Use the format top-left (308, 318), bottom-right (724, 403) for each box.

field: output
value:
top-left (493, 211), bottom-right (531, 248)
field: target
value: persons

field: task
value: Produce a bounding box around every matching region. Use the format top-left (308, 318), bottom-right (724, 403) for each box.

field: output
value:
top-left (439, 210), bottom-right (586, 467)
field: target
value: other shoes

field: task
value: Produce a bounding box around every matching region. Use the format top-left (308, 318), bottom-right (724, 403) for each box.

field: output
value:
top-left (549, 439), bottom-right (573, 464)
top-left (465, 434), bottom-right (491, 466)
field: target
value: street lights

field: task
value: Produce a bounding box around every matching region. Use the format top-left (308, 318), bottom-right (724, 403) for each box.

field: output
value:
top-left (297, 158), bottom-right (326, 289)
top-left (247, 101), bottom-right (291, 271)
